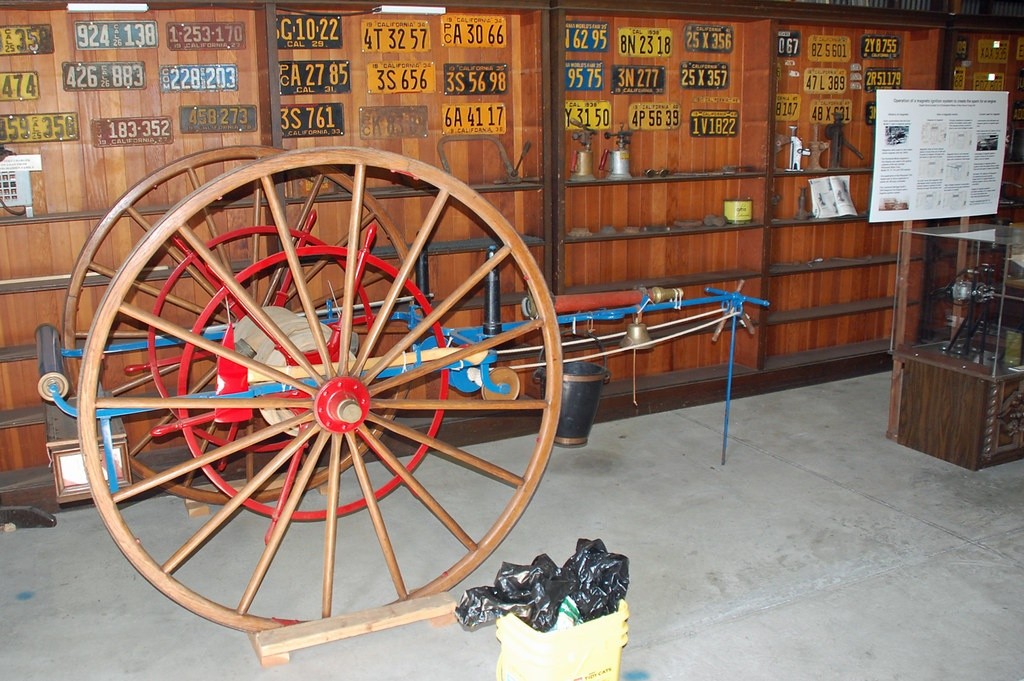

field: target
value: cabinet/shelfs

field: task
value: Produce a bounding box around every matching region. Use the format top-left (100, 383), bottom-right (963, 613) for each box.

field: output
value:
top-left (0, 0), bottom-right (1024, 526)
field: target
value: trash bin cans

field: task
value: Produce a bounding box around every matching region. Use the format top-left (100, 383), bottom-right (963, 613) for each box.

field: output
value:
top-left (497, 600), bottom-right (630, 681)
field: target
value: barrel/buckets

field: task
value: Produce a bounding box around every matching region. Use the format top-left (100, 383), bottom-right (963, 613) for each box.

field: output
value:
top-left (531, 330), bottom-right (611, 447)
top-left (723, 199), bottom-right (753, 225)
